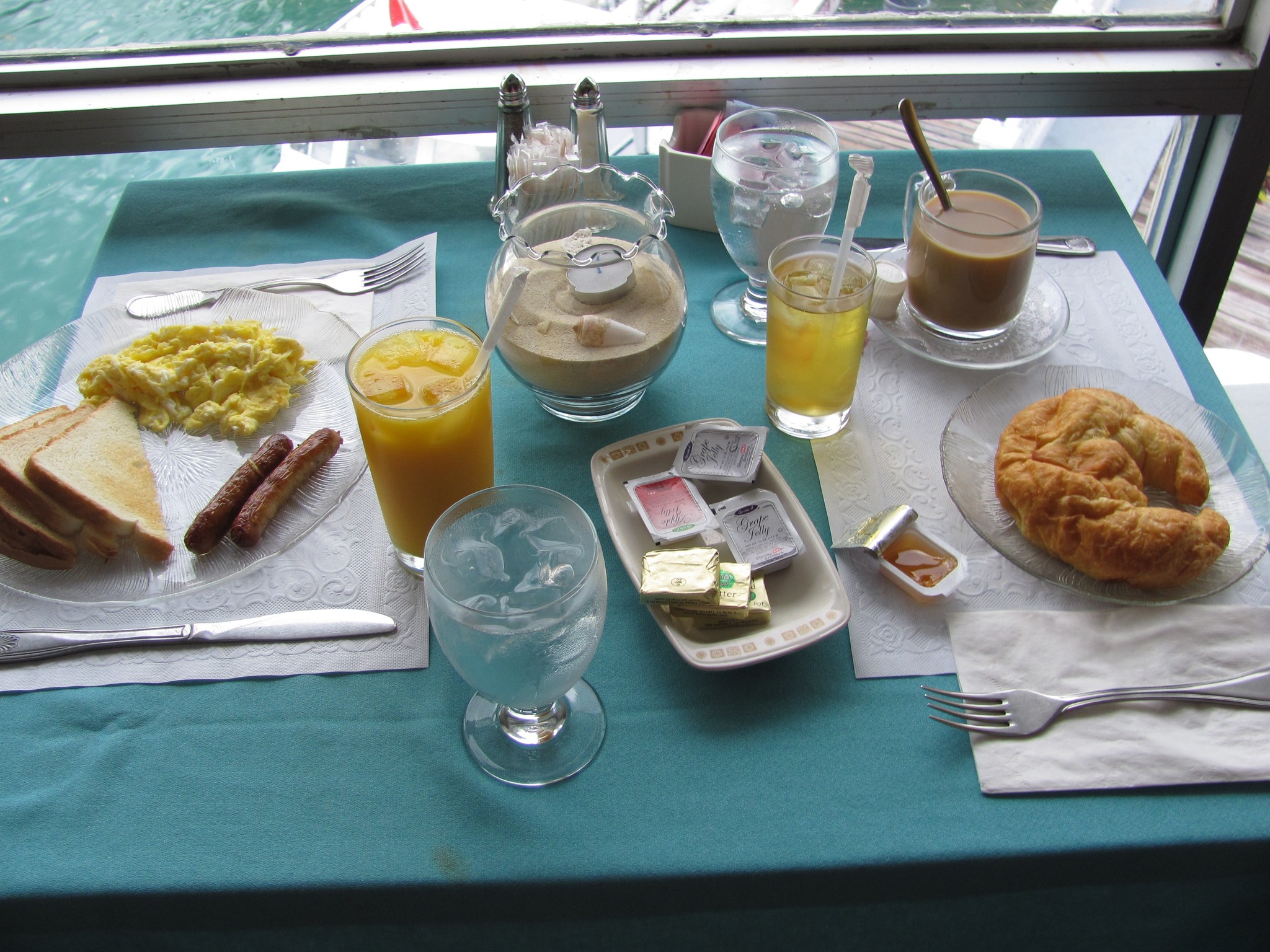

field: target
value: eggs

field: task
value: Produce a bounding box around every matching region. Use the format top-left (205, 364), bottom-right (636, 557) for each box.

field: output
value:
top-left (78, 316), bottom-right (321, 437)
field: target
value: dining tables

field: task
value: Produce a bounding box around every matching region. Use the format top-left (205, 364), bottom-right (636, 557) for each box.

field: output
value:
top-left (0, 143), bottom-right (1267, 950)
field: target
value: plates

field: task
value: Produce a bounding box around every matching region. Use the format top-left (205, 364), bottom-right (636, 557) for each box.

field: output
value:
top-left (938, 362), bottom-right (1268, 605)
top-left (868, 243), bottom-right (1071, 369)
top-left (1, 288), bottom-right (375, 606)
top-left (591, 415), bottom-right (852, 673)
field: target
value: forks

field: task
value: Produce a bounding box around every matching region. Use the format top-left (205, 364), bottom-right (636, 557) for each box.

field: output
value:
top-left (125, 237), bottom-right (426, 318)
top-left (920, 667), bottom-right (1270, 736)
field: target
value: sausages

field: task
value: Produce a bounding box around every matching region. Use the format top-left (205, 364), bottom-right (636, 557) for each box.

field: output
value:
top-left (183, 428), bottom-right (344, 555)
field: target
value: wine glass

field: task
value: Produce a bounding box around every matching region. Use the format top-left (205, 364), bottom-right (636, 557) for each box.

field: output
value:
top-left (423, 483), bottom-right (609, 789)
top-left (703, 104), bottom-right (842, 348)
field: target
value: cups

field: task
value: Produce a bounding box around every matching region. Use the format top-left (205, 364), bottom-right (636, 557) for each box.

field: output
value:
top-left (484, 163), bottom-right (687, 422)
top-left (763, 234), bottom-right (875, 440)
top-left (344, 316), bottom-right (495, 583)
top-left (902, 166), bottom-right (1044, 344)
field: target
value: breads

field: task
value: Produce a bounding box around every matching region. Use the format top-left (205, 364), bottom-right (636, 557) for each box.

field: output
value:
top-left (1, 397), bottom-right (174, 568)
top-left (992, 390), bottom-right (1230, 588)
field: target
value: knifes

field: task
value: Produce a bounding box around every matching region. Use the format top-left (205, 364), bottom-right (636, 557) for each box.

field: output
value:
top-left (1, 609), bottom-right (399, 676)
top-left (821, 234), bottom-right (1096, 259)
